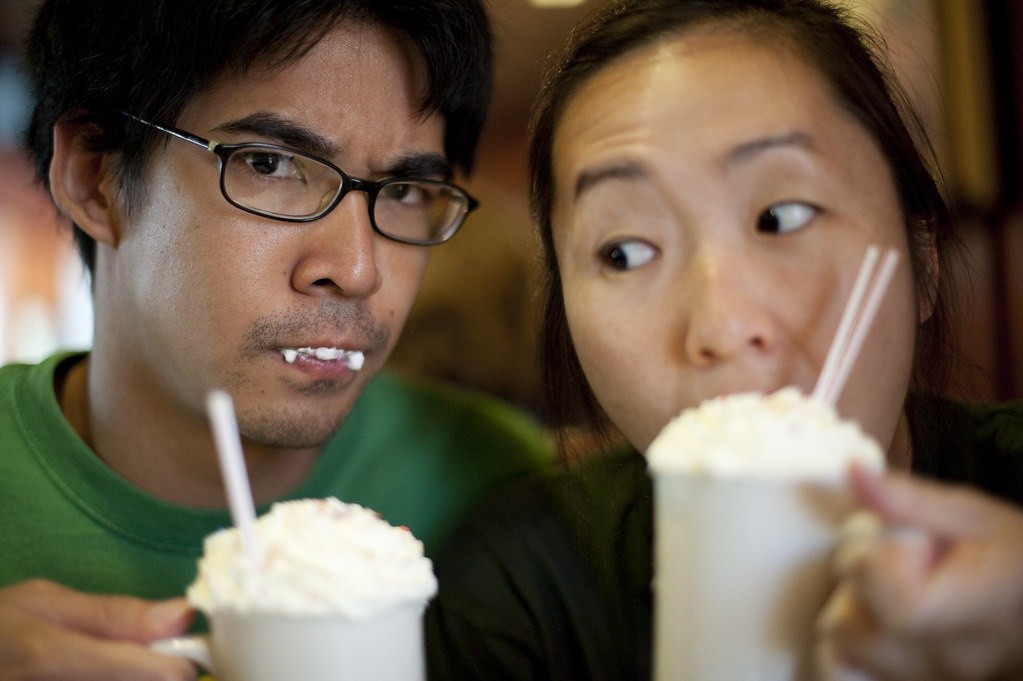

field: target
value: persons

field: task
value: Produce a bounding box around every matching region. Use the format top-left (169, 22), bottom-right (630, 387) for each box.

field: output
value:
top-left (0, 0), bottom-right (561, 681)
top-left (425, 0), bottom-right (1022, 681)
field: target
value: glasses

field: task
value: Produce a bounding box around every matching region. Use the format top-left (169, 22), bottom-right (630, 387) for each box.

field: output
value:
top-left (101, 104), bottom-right (481, 247)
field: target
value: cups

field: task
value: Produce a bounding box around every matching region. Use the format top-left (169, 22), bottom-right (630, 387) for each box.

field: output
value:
top-left (647, 463), bottom-right (889, 680)
top-left (149, 596), bottom-right (432, 681)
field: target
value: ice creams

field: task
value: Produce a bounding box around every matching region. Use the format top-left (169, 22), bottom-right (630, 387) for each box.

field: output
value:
top-left (182, 498), bottom-right (439, 681)
top-left (647, 382), bottom-right (886, 681)
